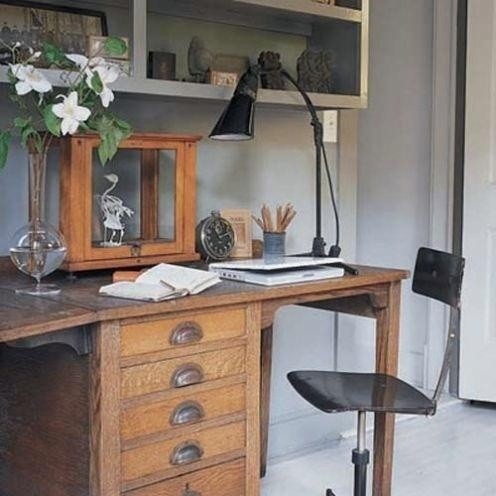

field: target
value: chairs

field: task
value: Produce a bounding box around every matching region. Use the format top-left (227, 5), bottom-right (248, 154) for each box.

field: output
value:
top-left (285, 247), bottom-right (466, 496)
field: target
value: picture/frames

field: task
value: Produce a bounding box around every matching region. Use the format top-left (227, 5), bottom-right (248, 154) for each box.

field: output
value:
top-left (220, 207), bottom-right (253, 258)
top-left (0, 0), bottom-right (108, 69)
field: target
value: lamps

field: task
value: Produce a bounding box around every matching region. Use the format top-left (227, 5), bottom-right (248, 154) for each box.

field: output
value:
top-left (208, 50), bottom-right (359, 275)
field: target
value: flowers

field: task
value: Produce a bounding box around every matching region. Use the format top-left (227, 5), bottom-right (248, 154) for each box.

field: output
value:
top-left (0, 36), bottom-right (137, 273)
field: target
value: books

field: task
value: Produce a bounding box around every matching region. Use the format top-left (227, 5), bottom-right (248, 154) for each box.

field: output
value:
top-left (99, 261), bottom-right (223, 303)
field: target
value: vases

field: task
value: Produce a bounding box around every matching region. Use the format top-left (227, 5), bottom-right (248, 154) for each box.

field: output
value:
top-left (7, 152), bottom-right (69, 294)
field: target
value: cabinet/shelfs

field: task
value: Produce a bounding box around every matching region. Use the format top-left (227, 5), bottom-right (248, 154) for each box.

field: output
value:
top-left (0, 0), bottom-right (370, 111)
top-left (25, 128), bottom-right (204, 282)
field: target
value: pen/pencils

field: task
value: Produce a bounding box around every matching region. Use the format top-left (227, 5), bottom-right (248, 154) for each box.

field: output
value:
top-left (160, 280), bottom-right (175, 291)
top-left (251, 202), bottom-right (296, 233)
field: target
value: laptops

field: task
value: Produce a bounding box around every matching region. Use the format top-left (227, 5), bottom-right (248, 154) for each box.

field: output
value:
top-left (208, 256), bottom-right (344, 286)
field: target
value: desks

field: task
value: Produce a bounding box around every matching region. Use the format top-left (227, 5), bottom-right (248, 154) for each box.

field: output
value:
top-left (0, 255), bottom-right (411, 496)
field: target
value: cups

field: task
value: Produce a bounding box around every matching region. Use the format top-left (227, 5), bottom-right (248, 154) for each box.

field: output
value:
top-left (263, 230), bottom-right (285, 264)
